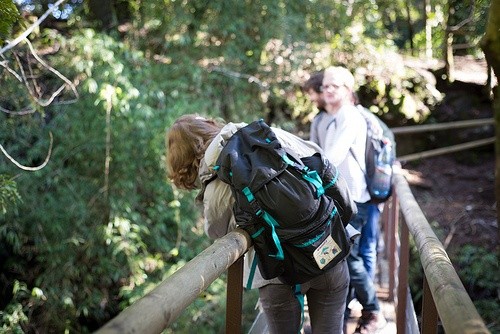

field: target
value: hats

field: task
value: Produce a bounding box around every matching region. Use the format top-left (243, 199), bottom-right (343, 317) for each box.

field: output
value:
top-left (322, 66), bottom-right (355, 92)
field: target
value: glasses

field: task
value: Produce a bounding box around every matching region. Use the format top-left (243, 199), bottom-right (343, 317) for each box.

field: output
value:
top-left (320, 83), bottom-right (342, 92)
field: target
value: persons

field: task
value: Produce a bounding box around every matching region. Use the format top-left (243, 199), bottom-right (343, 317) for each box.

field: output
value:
top-left (304, 66), bottom-right (397, 334)
top-left (168, 114), bottom-right (358, 334)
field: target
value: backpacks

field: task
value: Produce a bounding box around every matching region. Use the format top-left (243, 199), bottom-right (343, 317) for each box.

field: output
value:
top-left (327, 104), bottom-right (396, 204)
top-left (205, 119), bottom-right (352, 285)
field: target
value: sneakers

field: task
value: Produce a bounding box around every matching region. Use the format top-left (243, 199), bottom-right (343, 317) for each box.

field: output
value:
top-left (354, 310), bottom-right (386, 334)
